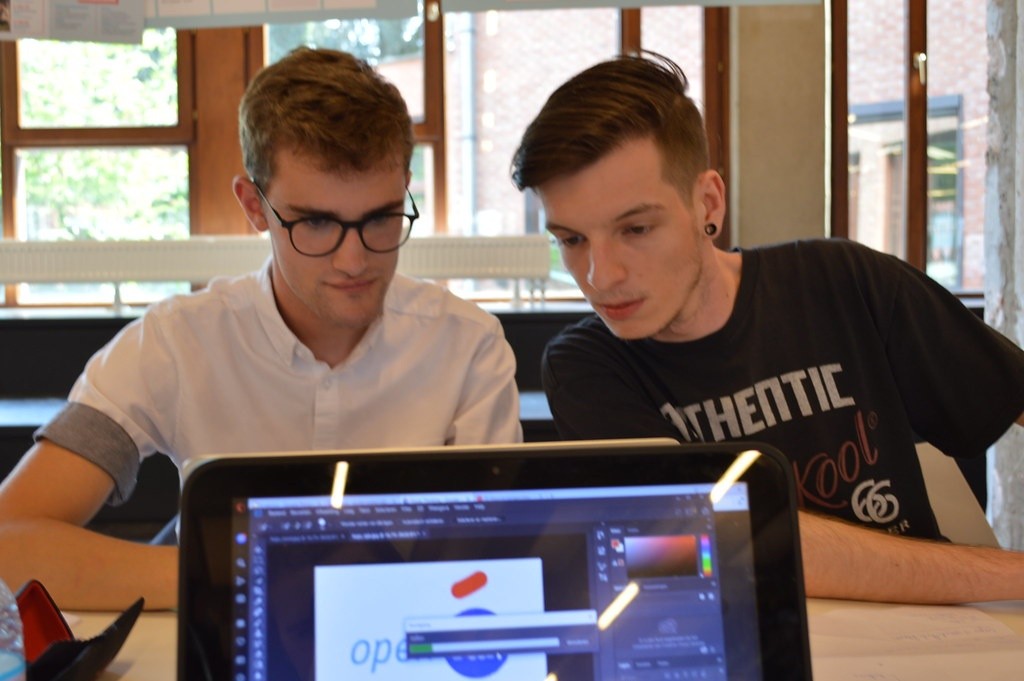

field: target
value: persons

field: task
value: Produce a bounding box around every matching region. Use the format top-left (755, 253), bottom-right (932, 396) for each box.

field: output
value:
top-left (509, 55), bottom-right (1024, 607)
top-left (0, 47), bottom-right (526, 615)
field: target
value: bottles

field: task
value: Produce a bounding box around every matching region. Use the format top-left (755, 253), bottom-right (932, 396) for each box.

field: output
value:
top-left (0, 578), bottom-right (28, 681)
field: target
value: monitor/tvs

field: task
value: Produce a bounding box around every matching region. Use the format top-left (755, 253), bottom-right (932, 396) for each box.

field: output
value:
top-left (177, 442), bottom-right (815, 681)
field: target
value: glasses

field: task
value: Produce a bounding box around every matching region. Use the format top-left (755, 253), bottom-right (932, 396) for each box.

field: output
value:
top-left (249, 175), bottom-right (419, 257)
